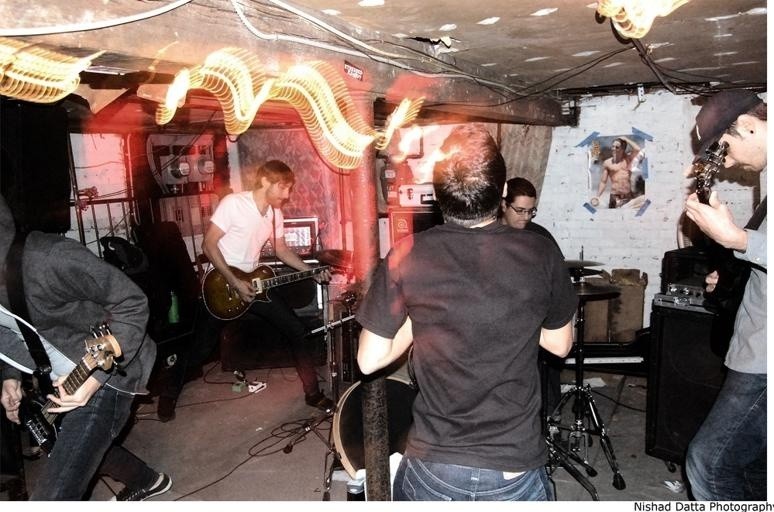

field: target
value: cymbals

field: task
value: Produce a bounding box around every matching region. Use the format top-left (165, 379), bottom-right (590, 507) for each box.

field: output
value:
top-left (314, 250), bottom-right (383, 271)
top-left (564, 260), bottom-right (621, 297)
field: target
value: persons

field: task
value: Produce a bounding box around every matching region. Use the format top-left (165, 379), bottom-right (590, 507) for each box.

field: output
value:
top-left (156, 159), bottom-right (340, 423)
top-left (494, 176), bottom-right (564, 256)
top-left (682, 83), bottom-right (766, 500)
top-left (592, 136), bottom-right (640, 212)
top-left (351, 120), bottom-right (584, 500)
top-left (0, 196), bottom-right (172, 499)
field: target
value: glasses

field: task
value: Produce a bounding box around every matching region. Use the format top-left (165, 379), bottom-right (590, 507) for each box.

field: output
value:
top-left (508, 202), bottom-right (537, 216)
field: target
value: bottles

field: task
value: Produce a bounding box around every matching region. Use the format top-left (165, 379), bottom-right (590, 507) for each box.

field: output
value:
top-left (167, 290), bottom-right (180, 324)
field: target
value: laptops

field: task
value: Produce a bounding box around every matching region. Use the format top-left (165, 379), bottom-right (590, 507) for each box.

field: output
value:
top-left (259, 217), bottom-right (319, 266)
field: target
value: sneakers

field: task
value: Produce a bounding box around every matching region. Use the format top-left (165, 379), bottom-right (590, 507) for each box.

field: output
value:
top-left (108, 472), bottom-right (172, 501)
top-left (306, 392), bottom-right (341, 415)
top-left (156, 389), bottom-right (179, 421)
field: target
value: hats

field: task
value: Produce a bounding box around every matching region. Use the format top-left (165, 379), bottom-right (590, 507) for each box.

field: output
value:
top-left (694, 85), bottom-right (762, 151)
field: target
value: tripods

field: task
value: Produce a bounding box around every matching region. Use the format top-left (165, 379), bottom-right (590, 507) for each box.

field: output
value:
top-left (543, 438), bottom-right (600, 501)
top-left (543, 299), bottom-right (626, 492)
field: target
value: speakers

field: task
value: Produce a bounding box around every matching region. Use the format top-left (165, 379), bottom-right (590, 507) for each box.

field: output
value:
top-left (645, 309), bottom-right (735, 465)
top-left (146, 322), bottom-right (203, 395)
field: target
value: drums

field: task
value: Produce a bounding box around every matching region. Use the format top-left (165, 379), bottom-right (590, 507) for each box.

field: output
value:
top-left (332, 352), bottom-right (414, 482)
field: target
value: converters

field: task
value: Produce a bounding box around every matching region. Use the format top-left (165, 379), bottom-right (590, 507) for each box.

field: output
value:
top-left (232, 381), bottom-right (246, 393)
top-left (249, 381), bottom-right (267, 394)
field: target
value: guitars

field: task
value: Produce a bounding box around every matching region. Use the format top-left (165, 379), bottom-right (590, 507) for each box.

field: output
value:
top-left (694, 141), bottom-right (751, 324)
top-left (18, 322), bottom-right (124, 455)
top-left (201, 264), bottom-right (350, 321)
